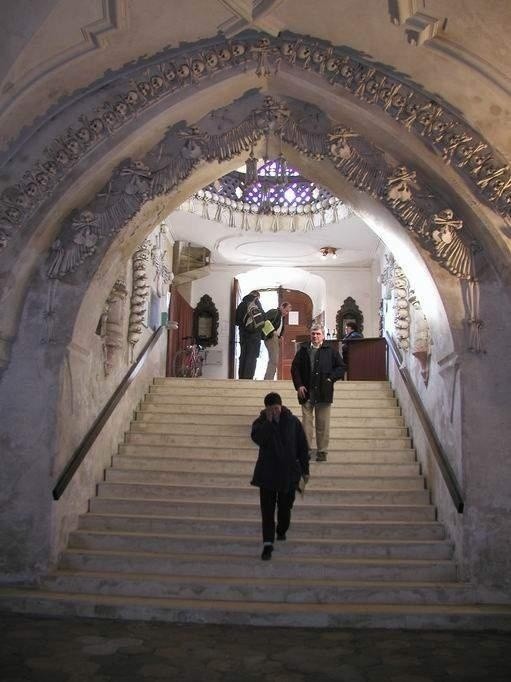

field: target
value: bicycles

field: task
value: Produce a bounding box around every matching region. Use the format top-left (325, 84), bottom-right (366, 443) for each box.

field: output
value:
top-left (172, 335), bottom-right (209, 378)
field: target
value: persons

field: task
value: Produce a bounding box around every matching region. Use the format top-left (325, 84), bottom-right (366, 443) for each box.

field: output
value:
top-left (290, 322), bottom-right (349, 464)
top-left (249, 391), bottom-right (313, 561)
top-left (261, 301), bottom-right (293, 381)
top-left (342, 321), bottom-right (365, 338)
top-left (235, 289), bottom-right (267, 381)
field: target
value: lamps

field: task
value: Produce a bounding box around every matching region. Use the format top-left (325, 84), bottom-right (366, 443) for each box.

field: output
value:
top-left (317, 244), bottom-right (339, 262)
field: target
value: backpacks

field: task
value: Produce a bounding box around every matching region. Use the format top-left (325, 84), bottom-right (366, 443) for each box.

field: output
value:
top-left (243, 297), bottom-right (265, 331)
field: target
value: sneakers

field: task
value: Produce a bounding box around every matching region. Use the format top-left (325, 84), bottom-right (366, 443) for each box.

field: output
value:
top-left (260, 545), bottom-right (273, 560)
top-left (276, 531), bottom-right (286, 541)
top-left (316, 451), bottom-right (327, 462)
